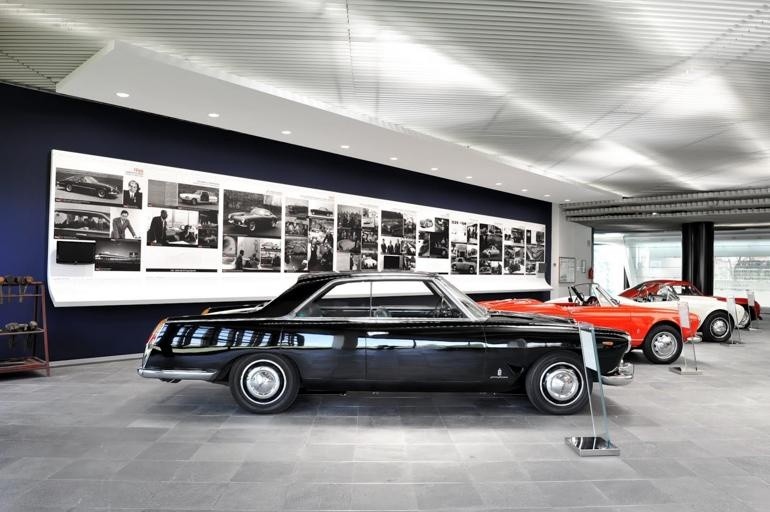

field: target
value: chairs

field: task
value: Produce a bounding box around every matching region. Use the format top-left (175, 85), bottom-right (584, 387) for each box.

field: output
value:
top-left (306, 303), bottom-right (345, 317)
top-left (374, 307), bottom-right (393, 318)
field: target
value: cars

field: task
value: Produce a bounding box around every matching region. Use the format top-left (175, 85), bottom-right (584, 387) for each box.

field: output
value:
top-left (137, 274), bottom-right (632, 416)
top-left (380, 221), bottom-right (502, 274)
top-left (227, 206), bottom-right (278, 232)
top-left (179, 190), bottom-right (218, 205)
top-left (57, 175), bottom-right (121, 199)
top-left (472, 280), bottom-right (701, 365)
top-left (618, 279), bottom-right (762, 341)
top-left (93, 238), bottom-right (140, 271)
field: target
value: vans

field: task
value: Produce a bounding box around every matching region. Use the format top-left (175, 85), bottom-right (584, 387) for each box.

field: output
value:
top-left (54, 209), bottom-right (111, 240)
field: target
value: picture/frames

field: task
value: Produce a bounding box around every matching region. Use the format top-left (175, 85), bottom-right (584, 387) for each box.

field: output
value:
top-left (559, 257), bottom-right (576, 283)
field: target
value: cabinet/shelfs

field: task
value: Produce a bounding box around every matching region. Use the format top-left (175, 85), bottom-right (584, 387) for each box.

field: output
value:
top-left (0, 279), bottom-right (51, 377)
